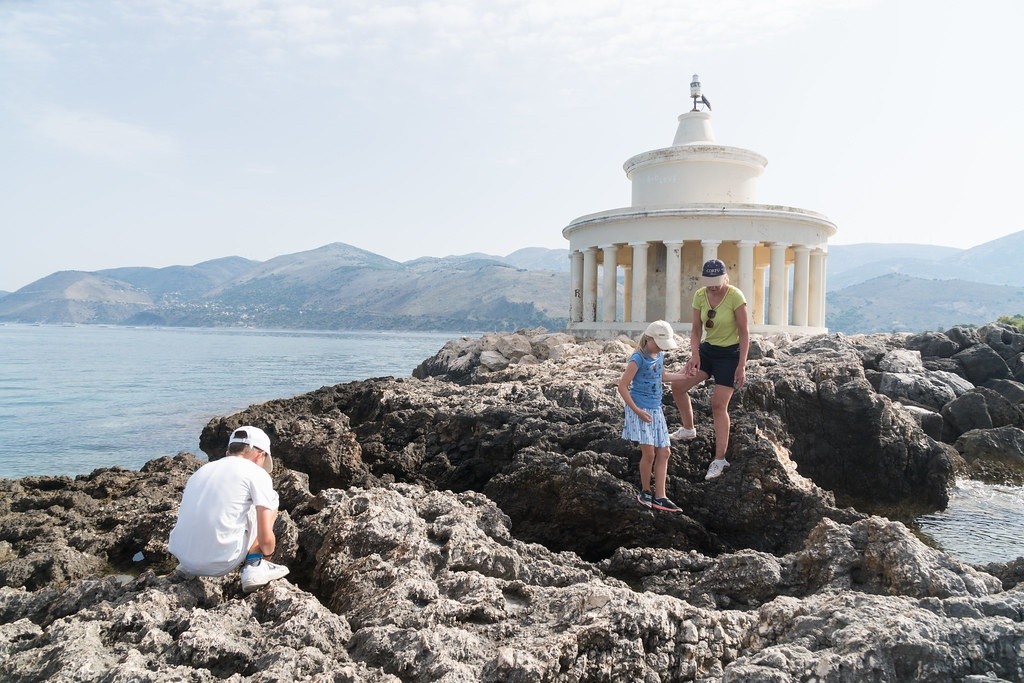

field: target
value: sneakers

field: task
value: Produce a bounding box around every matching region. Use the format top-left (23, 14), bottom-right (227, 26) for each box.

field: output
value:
top-left (240, 558), bottom-right (291, 593)
top-left (637, 490), bottom-right (653, 510)
top-left (705, 458), bottom-right (727, 480)
top-left (174, 563), bottom-right (197, 580)
top-left (653, 496), bottom-right (684, 514)
top-left (669, 428), bottom-right (696, 440)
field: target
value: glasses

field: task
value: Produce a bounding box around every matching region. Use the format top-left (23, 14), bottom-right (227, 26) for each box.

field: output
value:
top-left (706, 310), bottom-right (717, 328)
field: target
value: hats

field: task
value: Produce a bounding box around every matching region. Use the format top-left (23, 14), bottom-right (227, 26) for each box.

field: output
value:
top-left (701, 259), bottom-right (725, 286)
top-left (646, 319), bottom-right (678, 351)
top-left (229, 425), bottom-right (273, 472)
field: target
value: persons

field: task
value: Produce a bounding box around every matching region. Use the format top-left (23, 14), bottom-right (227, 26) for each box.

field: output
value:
top-left (618, 320), bottom-right (697, 512)
top-left (169, 426), bottom-right (290, 592)
top-left (669, 259), bottom-right (750, 481)
